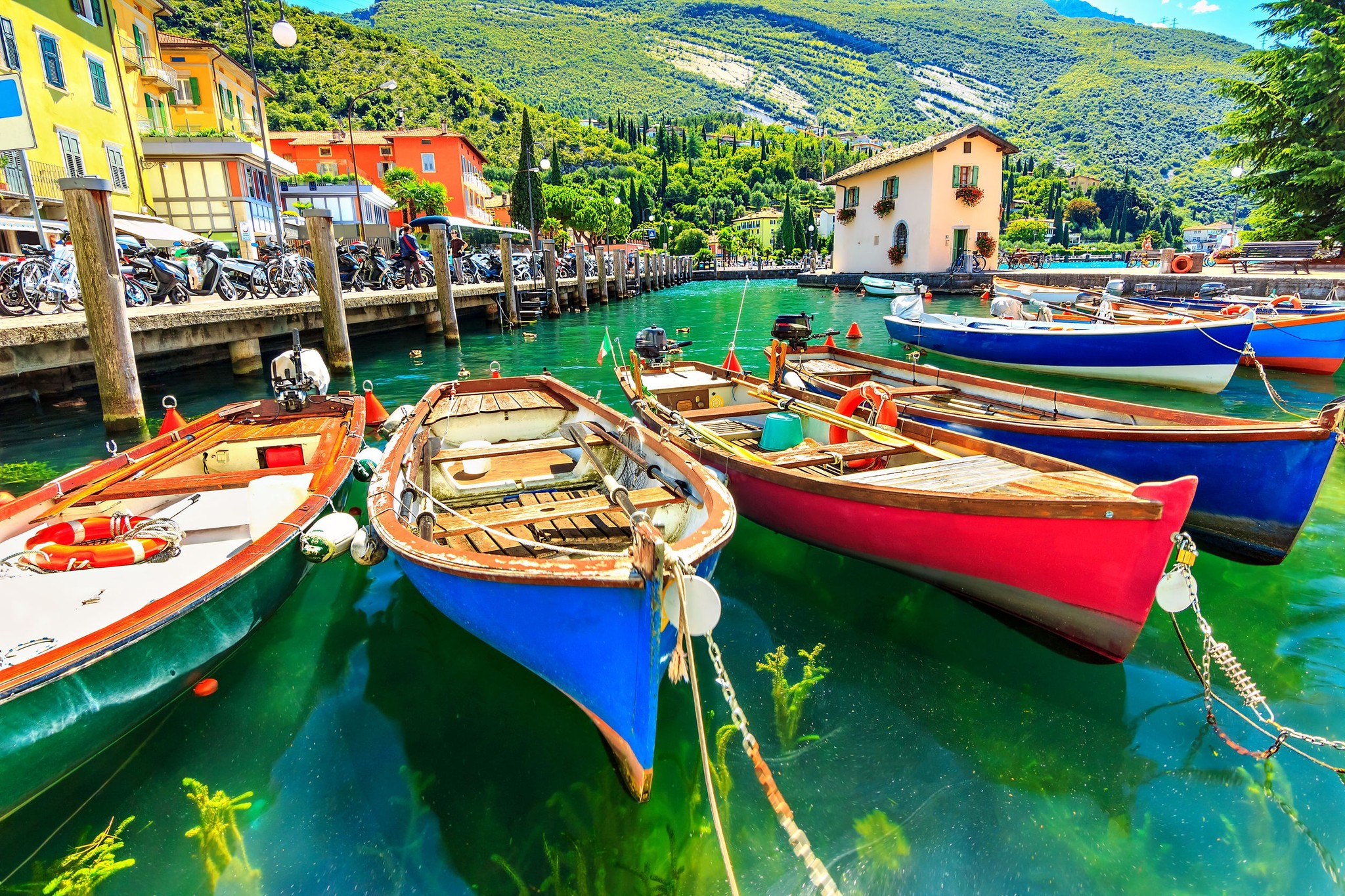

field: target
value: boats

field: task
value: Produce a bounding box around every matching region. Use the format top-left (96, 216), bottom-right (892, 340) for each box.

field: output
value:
top-left (0, 326), bottom-right (368, 896)
top-left (883, 308), bottom-right (1259, 396)
top-left (989, 275), bottom-right (1345, 377)
top-left (612, 322), bottom-right (1200, 665)
top-left (992, 274), bottom-right (1091, 306)
top-left (860, 276), bottom-right (929, 298)
top-left (760, 313), bottom-right (1344, 571)
top-left (365, 360), bottom-right (739, 806)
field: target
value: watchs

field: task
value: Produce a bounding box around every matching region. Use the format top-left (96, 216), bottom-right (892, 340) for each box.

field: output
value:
top-left (460, 249), bottom-right (462, 251)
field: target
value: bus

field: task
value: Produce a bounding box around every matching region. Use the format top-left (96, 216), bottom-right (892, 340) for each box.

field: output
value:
top-left (399, 215), bottom-right (534, 259)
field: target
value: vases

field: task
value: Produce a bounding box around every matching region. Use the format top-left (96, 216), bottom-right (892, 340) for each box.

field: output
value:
top-left (1215, 258), bottom-right (1240, 264)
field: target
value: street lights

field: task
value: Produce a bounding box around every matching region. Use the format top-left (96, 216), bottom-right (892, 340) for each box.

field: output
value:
top-left (642, 208), bottom-right (654, 253)
top-left (525, 142), bottom-right (551, 281)
top-left (240, 1), bottom-right (302, 298)
top-left (349, 80), bottom-right (398, 245)
top-left (762, 210), bottom-right (771, 246)
top-left (664, 220), bottom-right (673, 255)
top-left (1231, 157), bottom-right (1244, 231)
top-left (605, 187), bottom-right (620, 276)
top-left (509, 166), bottom-right (540, 228)
top-left (808, 225), bottom-right (814, 252)
top-left (573, 197), bottom-right (593, 245)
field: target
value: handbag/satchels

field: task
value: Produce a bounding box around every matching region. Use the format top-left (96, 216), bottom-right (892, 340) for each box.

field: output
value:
top-left (415, 250), bottom-right (426, 264)
top-left (459, 252), bottom-right (466, 256)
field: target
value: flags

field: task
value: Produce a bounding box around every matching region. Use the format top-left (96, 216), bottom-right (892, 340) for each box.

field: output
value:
top-left (597, 331), bottom-right (612, 365)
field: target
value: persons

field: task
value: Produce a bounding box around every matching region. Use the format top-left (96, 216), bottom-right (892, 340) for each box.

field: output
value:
top-left (450, 229), bottom-right (468, 285)
top-left (624, 254), bottom-right (627, 270)
top-left (727, 258), bottom-right (731, 267)
top-left (1219, 231), bottom-right (1235, 250)
top-left (742, 254), bottom-right (748, 267)
top-left (632, 249), bottom-right (640, 274)
top-left (766, 259), bottom-right (769, 266)
top-left (761, 259), bottom-right (767, 266)
top-left (1015, 248), bottom-right (1039, 269)
top-left (726, 259), bottom-right (728, 262)
top-left (770, 258), bottom-right (774, 266)
top-left (1140, 235), bottom-right (1153, 268)
top-left (810, 254), bottom-right (813, 258)
top-left (628, 249), bottom-right (633, 274)
top-left (794, 258), bottom-right (803, 265)
top-left (399, 223), bottom-right (428, 290)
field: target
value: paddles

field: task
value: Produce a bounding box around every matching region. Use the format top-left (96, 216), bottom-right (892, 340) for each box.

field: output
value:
top-left (904, 395), bottom-right (1051, 421)
top-left (582, 421), bottom-right (704, 510)
top-left (632, 388), bottom-right (771, 464)
top-left (559, 422), bottom-right (643, 525)
top-left (730, 376), bottom-right (962, 460)
top-left (1068, 286), bottom-right (1213, 322)
top-left (28, 420), bottom-right (228, 524)
top-left (996, 292), bottom-right (1142, 326)
top-left (307, 410), bottom-right (352, 492)
top-left (399, 427), bottom-right (432, 530)
top-left (416, 436), bottom-right (441, 543)
top-left (747, 390), bottom-right (914, 448)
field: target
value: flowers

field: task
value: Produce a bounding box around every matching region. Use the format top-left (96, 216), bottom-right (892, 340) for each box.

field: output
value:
top-left (872, 197), bottom-right (894, 220)
top-left (975, 235), bottom-right (997, 259)
top-left (885, 244), bottom-right (905, 268)
top-left (956, 186), bottom-right (984, 208)
top-left (1210, 247), bottom-right (1240, 260)
top-left (836, 207), bottom-right (855, 225)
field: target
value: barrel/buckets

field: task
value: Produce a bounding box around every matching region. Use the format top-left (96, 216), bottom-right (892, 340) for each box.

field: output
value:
top-left (757, 412), bottom-right (804, 451)
top-left (459, 440), bottom-right (492, 474)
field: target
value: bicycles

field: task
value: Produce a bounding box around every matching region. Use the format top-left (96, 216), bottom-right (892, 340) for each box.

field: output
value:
top-left (997, 248), bottom-right (1051, 270)
top-left (1202, 255), bottom-right (1216, 267)
top-left (0, 227), bottom-right (697, 318)
top-left (951, 247), bottom-right (987, 273)
top-left (1126, 250), bottom-right (1154, 268)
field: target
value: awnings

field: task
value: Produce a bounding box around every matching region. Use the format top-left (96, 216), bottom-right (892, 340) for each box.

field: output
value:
top-left (114, 215), bottom-right (214, 243)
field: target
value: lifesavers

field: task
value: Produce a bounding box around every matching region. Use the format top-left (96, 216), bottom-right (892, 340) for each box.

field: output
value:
top-left (1172, 255), bottom-right (1193, 273)
top-left (829, 381), bottom-right (898, 469)
top-left (1161, 317), bottom-right (1195, 325)
top-left (1216, 304), bottom-right (1251, 318)
top-left (1267, 295), bottom-right (1300, 308)
top-left (23, 516), bottom-right (172, 571)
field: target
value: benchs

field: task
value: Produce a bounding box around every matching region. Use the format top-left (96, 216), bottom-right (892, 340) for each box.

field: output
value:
top-left (1228, 240), bottom-right (1322, 274)
top-left (1124, 250), bottom-right (1161, 263)
top-left (764, 438), bottom-right (920, 469)
top-left (430, 485), bottom-right (687, 540)
top-left (889, 384), bottom-right (959, 396)
top-left (1012, 252), bottom-right (1044, 269)
top-left (677, 402), bottom-right (788, 423)
top-left (54, 464), bottom-right (318, 503)
top-left (415, 430), bottom-right (615, 464)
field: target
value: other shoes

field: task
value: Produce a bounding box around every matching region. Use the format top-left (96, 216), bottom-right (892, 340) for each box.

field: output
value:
top-left (407, 285), bottom-right (412, 290)
top-left (456, 283), bottom-right (463, 285)
top-left (421, 282), bottom-right (427, 288)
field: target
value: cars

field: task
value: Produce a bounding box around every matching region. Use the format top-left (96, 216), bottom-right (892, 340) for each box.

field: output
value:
top-left (716, 257), bottom-right (812, 268)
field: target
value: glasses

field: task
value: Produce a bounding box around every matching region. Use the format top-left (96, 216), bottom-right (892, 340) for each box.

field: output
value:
top-left (451, 232), bottom-right (456, 234)
